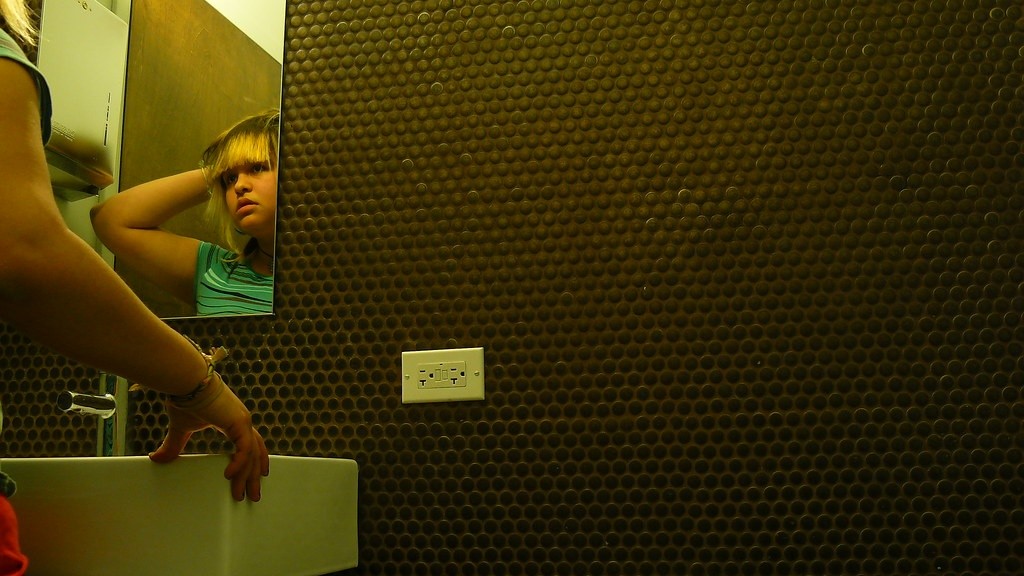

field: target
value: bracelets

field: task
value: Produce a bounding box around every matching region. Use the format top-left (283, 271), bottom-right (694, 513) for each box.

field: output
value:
top-left (202, 167), bottom-right (212, 197)
top-left (129, 335), bottom-right (228, 411)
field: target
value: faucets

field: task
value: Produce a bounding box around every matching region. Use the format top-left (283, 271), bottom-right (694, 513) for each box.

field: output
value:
top-left (55, 374), bottom-right (128, 457)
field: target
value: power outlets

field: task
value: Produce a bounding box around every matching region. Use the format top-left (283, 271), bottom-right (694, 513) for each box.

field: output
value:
top-left (402, 348), bottom-right (484, 404)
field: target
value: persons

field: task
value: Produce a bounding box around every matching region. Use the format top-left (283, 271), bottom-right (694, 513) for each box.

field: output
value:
top-left (90, 110), bottom-right (279, 316)
top-left (0, 0), bottom-right (268, 576)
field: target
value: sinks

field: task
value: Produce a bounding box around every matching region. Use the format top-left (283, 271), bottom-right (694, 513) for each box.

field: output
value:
top-left (0, 453), bottom-right (359, 576)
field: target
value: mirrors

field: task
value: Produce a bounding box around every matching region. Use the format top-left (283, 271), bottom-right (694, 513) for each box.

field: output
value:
top-left (19, 0), bottom-right (286, 319)
